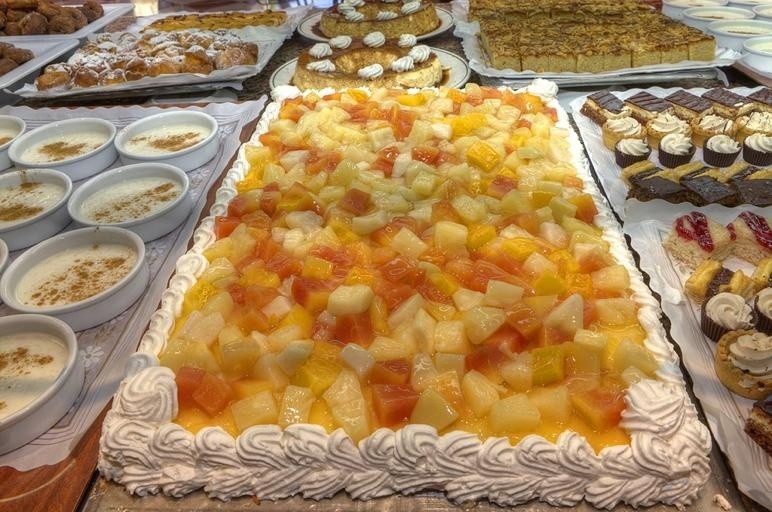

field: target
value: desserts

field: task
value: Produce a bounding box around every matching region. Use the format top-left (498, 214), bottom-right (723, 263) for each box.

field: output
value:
top-left (661, 210), bottom-right (772, 457)
top-left (579, 86), bottom-right (772, 208)
top-left (0, 122), bottom-right (212, 426)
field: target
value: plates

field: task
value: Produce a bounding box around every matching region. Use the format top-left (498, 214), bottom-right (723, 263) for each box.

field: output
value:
top-left (273, 1), bottom-right (474, 92)
top-left (1, 314), bottom-right (87, 458)
top-left (8, 117), bottom-right (118, 183)
top-left (0, 226), bottom-right (151, 334)
top-left (1, 169), bottom-right (72, 252)
top-left (69, 162), bottom-right (192, 244)
top-left (0, 114), bottom-right (26, 172)
top-left (113, 111), bottom-right (222, 173)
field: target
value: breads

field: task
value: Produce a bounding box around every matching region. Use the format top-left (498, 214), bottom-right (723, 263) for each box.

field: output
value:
top-left (0, 1), bottom-right (292, 91)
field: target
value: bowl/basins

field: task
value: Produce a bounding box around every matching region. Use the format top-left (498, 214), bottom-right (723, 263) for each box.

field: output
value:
top-left (658, 2), bottom-right (770, 76)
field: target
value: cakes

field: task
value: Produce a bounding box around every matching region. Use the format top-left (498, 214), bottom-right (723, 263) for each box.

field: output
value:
top-left (292, 32), bottom-right (444, 90)
top-left (318, 0), bottom-right (440, 39)
top-left (663, 1), bottom-right (772, 55)
top-left (467, 0), bottom-right (717, 73)
top-left (99, 79), bottom-right (712, 508)
